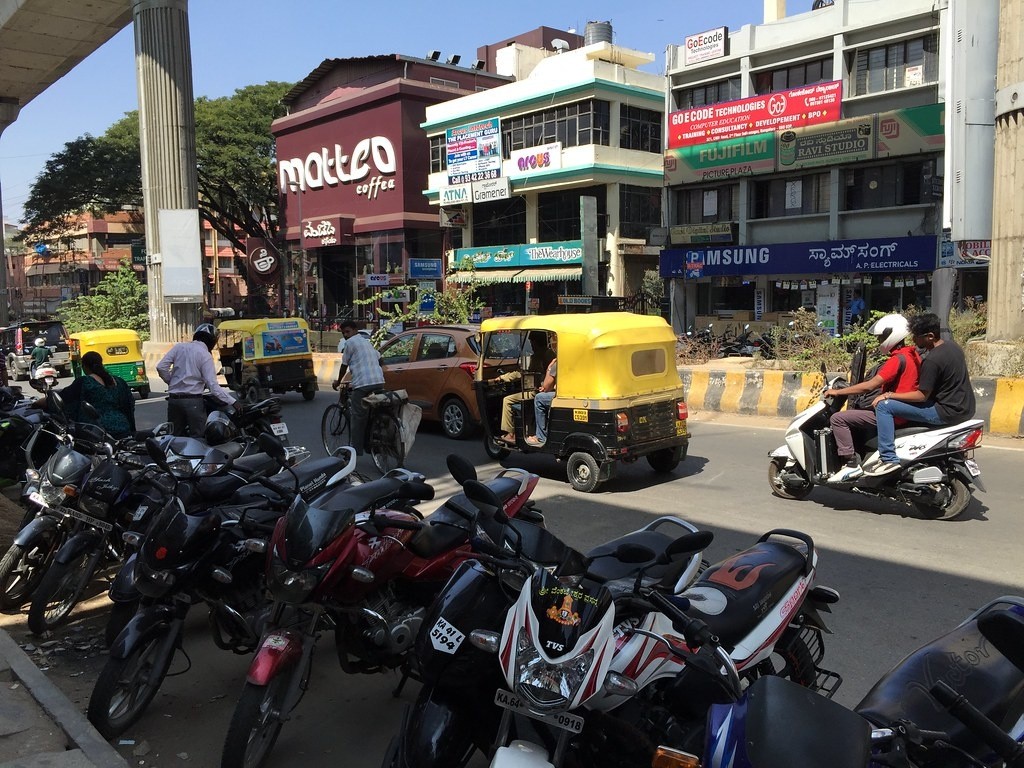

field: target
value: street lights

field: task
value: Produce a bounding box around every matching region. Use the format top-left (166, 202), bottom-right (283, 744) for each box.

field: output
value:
top-left (287, 181), bottom-right (309, 317)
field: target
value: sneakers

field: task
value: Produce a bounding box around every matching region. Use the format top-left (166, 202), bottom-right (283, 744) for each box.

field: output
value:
top-left (827, 464), bottom-right (864, 482)
top-left (864, 460), bottom-right (902, 476)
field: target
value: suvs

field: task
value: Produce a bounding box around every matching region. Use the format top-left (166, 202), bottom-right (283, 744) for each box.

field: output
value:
top-left (0, 320), bottom-right (72, 381)
top-left (343, 324), bottom-right (481, 439)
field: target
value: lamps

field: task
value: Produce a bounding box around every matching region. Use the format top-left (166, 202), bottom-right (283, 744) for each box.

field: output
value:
top-left (446, 54), bottom-right (461, 65)
top-left (471, 59), bottom-right (485, 70)
top-left (426, 49), bottom-right (441, 61)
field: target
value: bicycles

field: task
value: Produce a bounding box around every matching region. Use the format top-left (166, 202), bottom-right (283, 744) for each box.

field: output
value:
top-left (320, 381), bottom-right (407, 476)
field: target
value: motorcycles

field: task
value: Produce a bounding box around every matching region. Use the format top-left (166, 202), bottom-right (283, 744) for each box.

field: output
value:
top-left (634, 590), bottom-right (1024, 768)
top-left (217, 318), bottom-right (319, 402)
top-left (0, 375), bottom-right (843, 768)
top-left (470, 312), bottom-right (692, 494)
top-left (66, 327), bottom-right (151, 399)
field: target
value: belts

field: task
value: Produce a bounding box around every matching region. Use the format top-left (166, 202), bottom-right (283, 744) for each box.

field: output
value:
top-left (170, 394), bottom-right (202, 399)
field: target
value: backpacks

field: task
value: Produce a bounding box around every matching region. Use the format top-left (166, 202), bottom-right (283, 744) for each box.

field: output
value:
top-left (850, 354), bottom-right (906, 410)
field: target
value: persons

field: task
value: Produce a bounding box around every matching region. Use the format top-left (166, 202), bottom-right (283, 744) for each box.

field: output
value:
top-left (840, 289), bottom-right (865, 326)
top-left (332, 320), bottom-right (385, 456)
top-left (28, 337), bottom-right (53, 384)
top-left (0, 345), bottom-right (8, 387)
top-left (821, 312), bottom-right (923, 481)
top-left (32, 352), bottom-right (135, 440)
top-left (527, 357), bottom-right (557, 444)
top-left (863, 313), bottom-right (976, 476)
top-left (494, 331), bottom-right (556, 445)
top-left (156, 323), bottom-right (244, 438)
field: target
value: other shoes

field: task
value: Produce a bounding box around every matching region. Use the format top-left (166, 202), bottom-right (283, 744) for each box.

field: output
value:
top-left (494, 434), bottom-right (516, 445)
top-left (526, 435), bottom-right (545, 448)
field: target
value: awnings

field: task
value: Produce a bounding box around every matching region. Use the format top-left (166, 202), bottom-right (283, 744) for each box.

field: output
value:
top-left (513, 266), bottom-right (582, 283)
top-left (445, 269), bottom-right (522, 283)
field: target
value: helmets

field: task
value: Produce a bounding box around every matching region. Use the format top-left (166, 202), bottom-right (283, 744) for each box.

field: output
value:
top-left (193, 323), bottom-right (220, 351)
top-left (206, 411), bottom-right (234, 445)
top-left (874, 314), bottom-right (912, 355)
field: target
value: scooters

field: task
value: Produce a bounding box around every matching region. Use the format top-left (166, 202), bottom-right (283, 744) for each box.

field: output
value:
top-left (768, 361), bottom-right (987, 521)
top-left (686, 323), bottom-right (785, 359)
top-left (29, 361), bottom-right (59, 395)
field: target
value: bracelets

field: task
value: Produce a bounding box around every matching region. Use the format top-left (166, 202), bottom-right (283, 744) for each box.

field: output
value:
top-left (547, 368), bottom-right (551, 371)
top-left (884, 391), bottom-right (893, 398)
